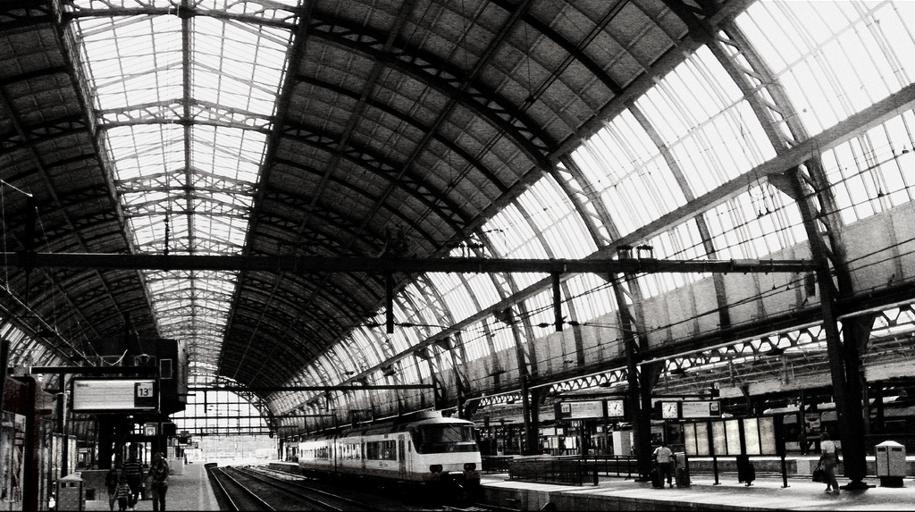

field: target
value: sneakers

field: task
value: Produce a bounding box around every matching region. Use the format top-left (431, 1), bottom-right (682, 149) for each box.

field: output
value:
top-left (824, 488), bottom-right (839, 493)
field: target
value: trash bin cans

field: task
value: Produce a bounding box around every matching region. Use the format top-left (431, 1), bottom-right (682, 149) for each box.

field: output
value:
top-left (57, 475), bottom-right (86, 511)
top-left (875, 440), bottom-right (906, 488)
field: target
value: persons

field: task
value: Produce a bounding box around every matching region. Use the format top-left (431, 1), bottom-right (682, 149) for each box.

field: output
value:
top-left (109, 472), bottom-right (132, 511)
top-left (103, 469), bottom-right (119, 511)
top-left (160, 451), bottom-right (170, 473)
top-left (141, 452), bottom-right (171, 511)
top-left (651, 440), bottom-right (676, 488)
top-left (119, 449), bottom-right (144, 510)
top-left (816, 430), bottom-right (843, 495)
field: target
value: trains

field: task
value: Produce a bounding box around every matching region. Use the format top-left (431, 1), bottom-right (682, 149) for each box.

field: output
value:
top-left (295, 417), bottom-right (483, 489)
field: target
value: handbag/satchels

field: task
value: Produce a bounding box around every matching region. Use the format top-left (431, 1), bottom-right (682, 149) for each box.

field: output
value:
top-left (812, 470), bottom-right (825, 482)
